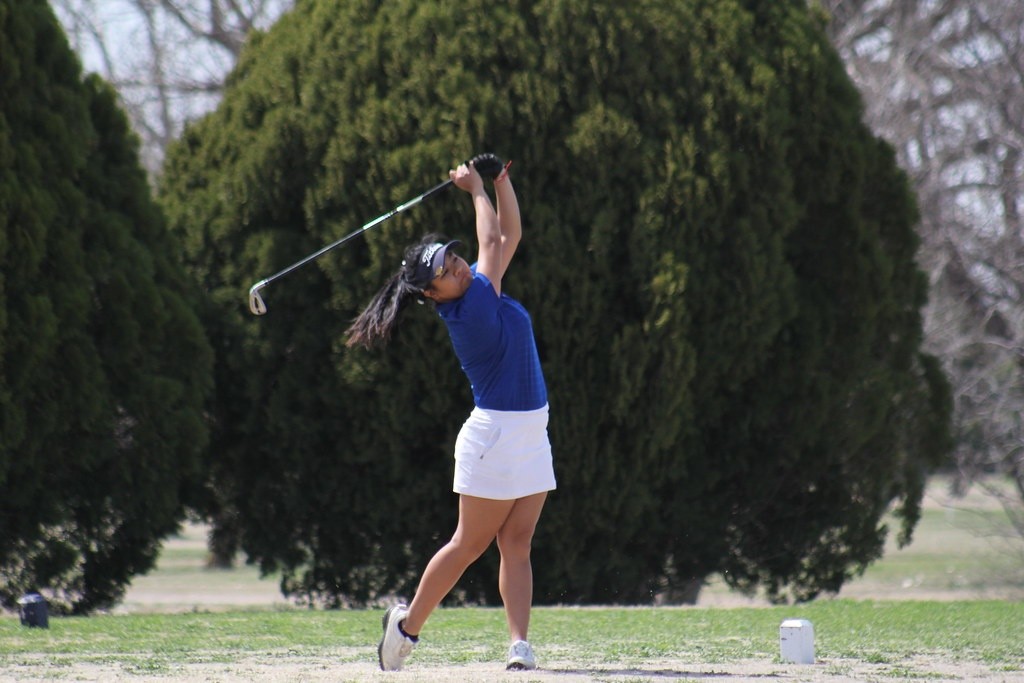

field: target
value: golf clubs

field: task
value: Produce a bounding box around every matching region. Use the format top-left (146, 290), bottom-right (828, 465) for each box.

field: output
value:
top-left (250, 154), bottom-right (500, 315)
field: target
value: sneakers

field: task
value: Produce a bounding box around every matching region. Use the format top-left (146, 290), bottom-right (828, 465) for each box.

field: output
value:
top-left (507, 640), bottom-right (536, 669)
top-left (377, 603), bottom-right (416, 672)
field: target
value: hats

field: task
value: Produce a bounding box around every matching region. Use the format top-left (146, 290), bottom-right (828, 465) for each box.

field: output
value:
top-left (418, 240), bottom-right (462, 280)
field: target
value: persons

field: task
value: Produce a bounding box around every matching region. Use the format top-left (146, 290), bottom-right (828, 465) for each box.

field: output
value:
top-left (343, 160), bottom-right (559, 676)
